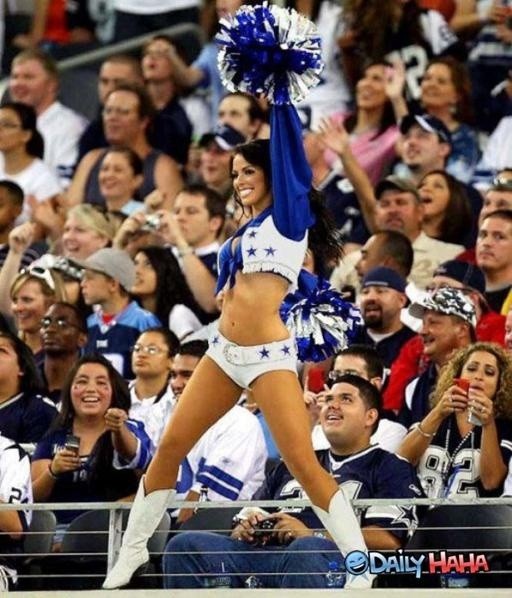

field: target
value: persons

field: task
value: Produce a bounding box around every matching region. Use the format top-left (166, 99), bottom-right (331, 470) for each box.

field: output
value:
top-left (0, 0), bottom-right (512, 593)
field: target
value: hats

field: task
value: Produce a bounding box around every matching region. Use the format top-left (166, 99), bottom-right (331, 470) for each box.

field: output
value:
top-left (410, 260), bottom-right (485, 328)
top-left (198, 123), bottom-right (245, 151)
top-left (68, 248), bottom-right (136, 292)
top-left (363, 267), bottom-right (411, 307)
top-left (30, 248), bottom-right (80, 292)
top-left (399, 114), bottom-right (451, 142)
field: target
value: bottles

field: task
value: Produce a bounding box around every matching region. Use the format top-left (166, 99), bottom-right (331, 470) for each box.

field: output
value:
top-left (326, 561), bottom-right (344, 588)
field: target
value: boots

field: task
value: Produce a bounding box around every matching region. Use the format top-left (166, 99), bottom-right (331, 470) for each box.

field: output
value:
top-left (101, 474), bottom-right (177, 589)
top-left (311, 488), bottom-right (378, 588)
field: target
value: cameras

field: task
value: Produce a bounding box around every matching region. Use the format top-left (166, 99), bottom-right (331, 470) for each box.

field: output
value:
top-left (252, 516), bottom-right (278, 538)
top-left (138, 214), bottom-right (161, 232)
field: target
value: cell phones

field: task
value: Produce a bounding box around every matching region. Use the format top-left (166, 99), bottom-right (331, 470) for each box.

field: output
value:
top-left (64, 434), bottom-right (81, 458)
top-left (467, 399), bottom-right (484, 427)
top-left (451, 378), bottom-right (470, 411)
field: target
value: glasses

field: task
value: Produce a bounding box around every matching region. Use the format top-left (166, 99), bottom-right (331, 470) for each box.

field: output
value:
top-left (21, 266), bottom-right (55, 290)
top-left (132, 344), bottom-right (160, 354)
top-left (42, 318), bottom-right (68, 328)
